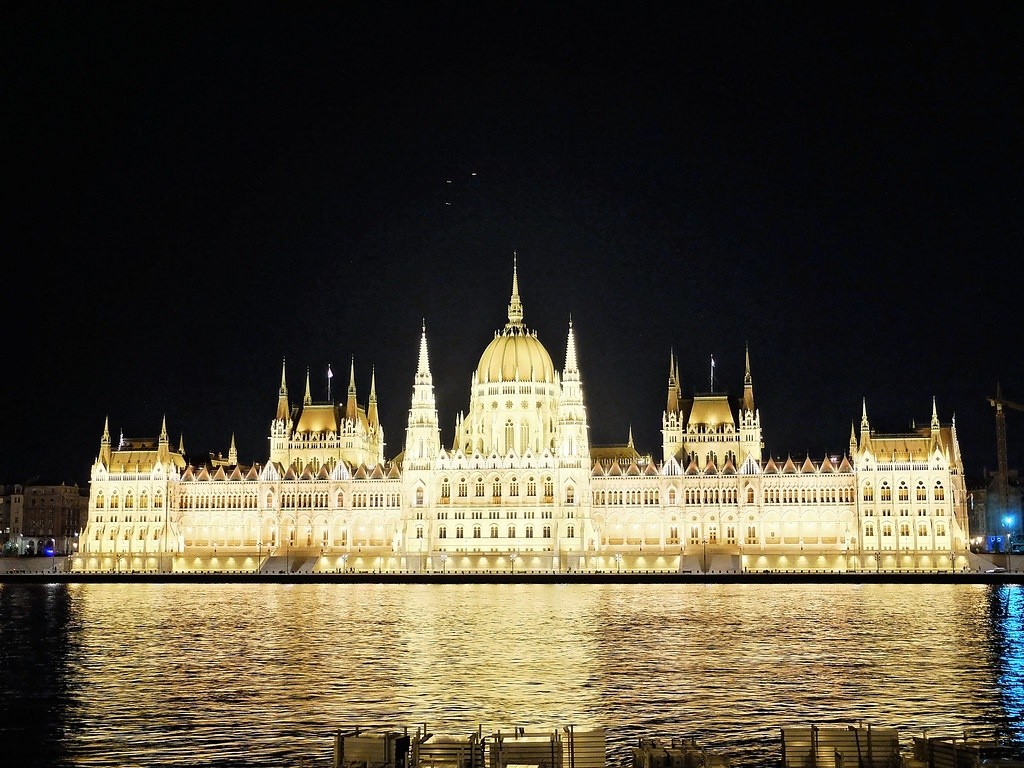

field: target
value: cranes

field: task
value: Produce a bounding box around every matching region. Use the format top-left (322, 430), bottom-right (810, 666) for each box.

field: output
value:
top-left (985, 382), bottom-right (1024, 532)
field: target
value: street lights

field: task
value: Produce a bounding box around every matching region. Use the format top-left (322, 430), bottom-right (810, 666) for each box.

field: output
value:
top-left (950, 552), bottom-right (956, 572)
top-left (68, 554), bottom-right (72, 572)
top-left (52, 539), bottom-right (56, 572)
top-left (874, 551), bottom-right (880, 571)
top-left (116, 554), bottom-right (121, 573)
top-left (441, 554), bottom-right (447, 574)
top-left (510, 554), bottom-right (515, 573)
top-left (616, 554), bottom-right (622, 574)
